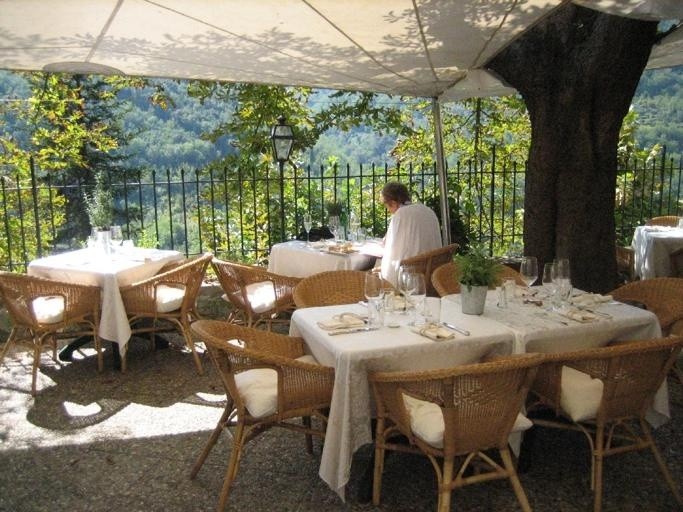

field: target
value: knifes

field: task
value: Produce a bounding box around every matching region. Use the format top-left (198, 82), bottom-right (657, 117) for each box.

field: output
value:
top-left (581, 306), bottom-right (612, 319)
top-left (328, 327), bottom-right (367, 336)
top-left (438, 321), bottom-right (469, 336)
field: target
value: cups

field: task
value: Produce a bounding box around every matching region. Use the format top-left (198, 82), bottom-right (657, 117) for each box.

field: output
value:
top-left (422, 294), bottom-right (441, 330)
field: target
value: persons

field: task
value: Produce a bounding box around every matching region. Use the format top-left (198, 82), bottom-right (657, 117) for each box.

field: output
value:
top-left (368, 181), bottom-right (442, 291)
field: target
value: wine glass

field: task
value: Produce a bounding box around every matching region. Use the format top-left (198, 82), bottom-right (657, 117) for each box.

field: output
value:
top-left (363, 262), bottom-right (427, 331)
top-left (303, 211), bottom-right (374, 256)
top-left (85, 223), bottom-right (123, 266)
top-left (494, 255), bottom-right (573, 315)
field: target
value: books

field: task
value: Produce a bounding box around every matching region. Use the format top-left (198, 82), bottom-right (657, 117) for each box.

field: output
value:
top-left (360, 239), bottom-right (385, 258)
